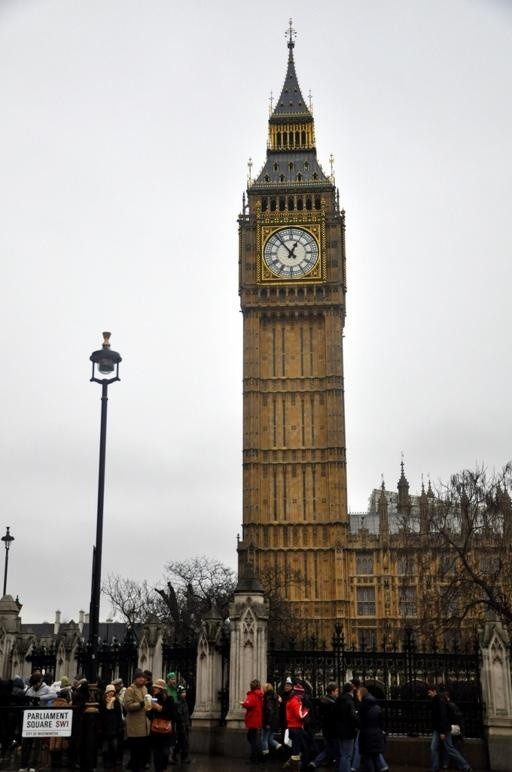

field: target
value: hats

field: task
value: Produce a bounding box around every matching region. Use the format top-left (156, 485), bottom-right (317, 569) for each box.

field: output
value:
top-left (293, 683), bottom-right (305, 692)
top-left (105, 685), bottom-right (116, 694)
top-left (176, 685), bottom-right (186, 693)
top-left (60, 676), bottom-right (71, 688)
top-left (167, 672), bottom-right (175, 679)
top-left (152, 679), bottom-right (166, 688)
top-left (133, 668), bottom-right (152, 681)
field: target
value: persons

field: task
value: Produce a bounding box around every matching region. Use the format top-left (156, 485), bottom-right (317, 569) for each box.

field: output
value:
top-left (239, 676), bottom-right (475, 772)
top-left (1, 666), bottom-right (193, 772)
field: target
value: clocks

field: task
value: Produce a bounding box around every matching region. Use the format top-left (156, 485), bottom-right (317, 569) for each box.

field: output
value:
top-left (254, 219), bottom-right (327, 287)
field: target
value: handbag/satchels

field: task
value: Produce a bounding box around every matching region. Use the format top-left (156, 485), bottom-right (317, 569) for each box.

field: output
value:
top-left (151, 718), bottom-right (173, 734)
top-left (451, 724), bottom-right (460, 735)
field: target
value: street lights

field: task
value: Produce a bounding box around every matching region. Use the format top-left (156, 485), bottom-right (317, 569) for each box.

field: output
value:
top-left (0, 523), bottom-right (14, 597)
top-left (84, 326), bottom-right (124, 681)
